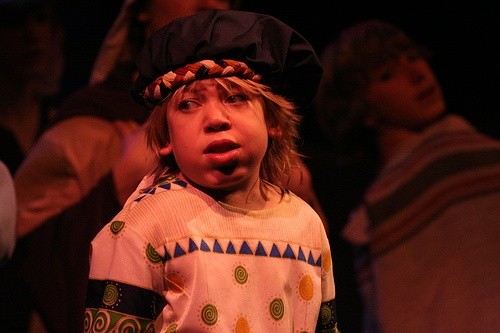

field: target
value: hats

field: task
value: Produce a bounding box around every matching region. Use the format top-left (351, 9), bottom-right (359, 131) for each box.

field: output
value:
top-left (313, 21), bottom-right (413, 133)
top-left (131, 9), bottom-right (322, 124)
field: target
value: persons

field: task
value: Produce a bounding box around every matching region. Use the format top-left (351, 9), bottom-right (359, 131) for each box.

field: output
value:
top-left (0, 0), bottom-right (500, 333)
top-left (81, 8), bottom-right (342, 333)
